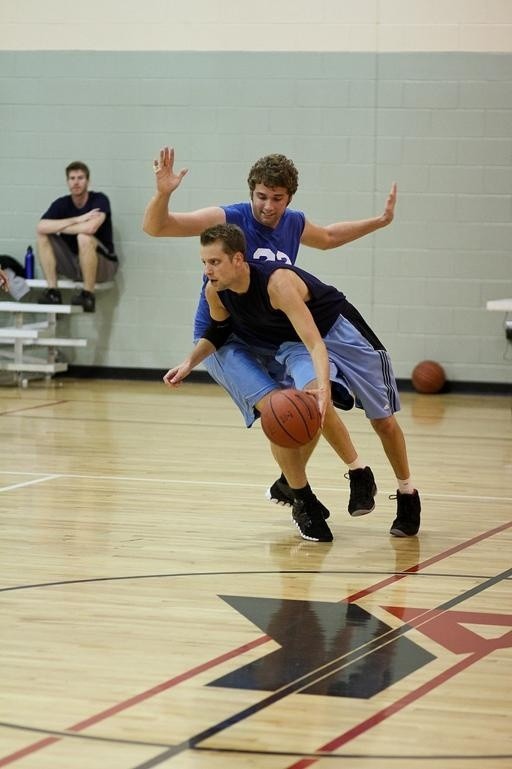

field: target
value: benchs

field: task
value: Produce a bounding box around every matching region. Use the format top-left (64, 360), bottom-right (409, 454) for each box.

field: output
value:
top-left (0, 277), bottom-right (116, 387)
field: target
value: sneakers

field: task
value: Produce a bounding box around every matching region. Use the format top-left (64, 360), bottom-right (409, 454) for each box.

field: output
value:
top-left (270, 479), bottom-right (333, 542)
top-left (71, 291), bottom-right (94, 313)
top-left (344, 466), bottom-right (377, 516)
top-left (37, 287), bottom-right (62, 303)
top-left (389, 489), bottom-right (421, 537)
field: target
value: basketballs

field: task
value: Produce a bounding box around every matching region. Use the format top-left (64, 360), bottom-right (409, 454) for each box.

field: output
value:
top-left (411, 360), bottom-right (445, 394)
top-left (261, 389), bottom-right (321, 448)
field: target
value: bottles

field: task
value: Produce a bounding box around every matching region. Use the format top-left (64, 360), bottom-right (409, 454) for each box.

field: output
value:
top-left (25, 244), bottom-right (34, 280)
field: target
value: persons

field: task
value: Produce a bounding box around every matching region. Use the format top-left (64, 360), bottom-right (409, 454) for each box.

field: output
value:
top-left (163, 223), bottom-right (422, 539)
top-left (142, 146), bottom-right (398, 544)
top-left (36, 161), bottom-right (120, 313)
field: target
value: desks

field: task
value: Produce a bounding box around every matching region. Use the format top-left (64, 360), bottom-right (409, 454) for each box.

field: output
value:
top-left (485, 297), bottom-right (512, 342)
